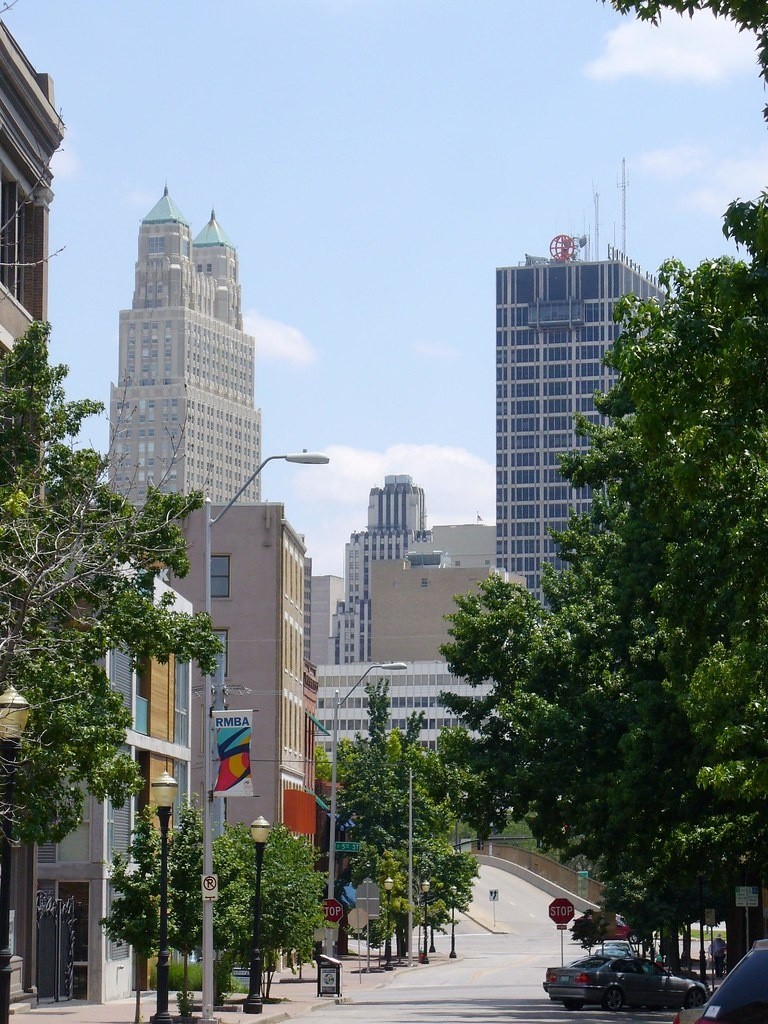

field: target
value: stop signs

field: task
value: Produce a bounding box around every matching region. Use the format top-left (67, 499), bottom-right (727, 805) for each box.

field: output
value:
top-left (320, 898), bottom-right (344, 925)
top-left (549, 897), bottom-right (575, 923)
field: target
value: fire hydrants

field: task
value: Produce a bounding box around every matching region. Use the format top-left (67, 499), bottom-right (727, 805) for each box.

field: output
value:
top-left (418, 947), bottom-right (424, 963)
top-left (656, 953), bottom-right (663, 968)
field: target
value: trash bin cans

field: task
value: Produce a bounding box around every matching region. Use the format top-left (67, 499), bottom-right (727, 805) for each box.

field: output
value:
top-left (316, 954), bottom-right (344, 998)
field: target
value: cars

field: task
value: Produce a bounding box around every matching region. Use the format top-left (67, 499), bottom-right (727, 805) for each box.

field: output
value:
top-left (594, 940), bottom-right (638, 958)
top-left (579, 910), bottom-right (644, 945)
top-left (672, 937), bottom-right (768, 1024)
top-left (542, 953), bottom-right (710, 1012)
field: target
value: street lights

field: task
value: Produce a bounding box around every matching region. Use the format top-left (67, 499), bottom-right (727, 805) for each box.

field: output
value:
top-left (0, 683), bottom-right (30, 1024)
top-left (195, 452), bottom-right (330, 1024)
top-left (325, 661), bottom-right (408, 961)
top-left (448, 884), bottom-right (457, 959)
top-left (381, 872), bottom-right (395, 971)
top-left (150, 768), bottom-right (175, 1024)
top-left (243, 809), bottom-right (272, 1015)
top-left (420, 878), bottom-right (430, 964)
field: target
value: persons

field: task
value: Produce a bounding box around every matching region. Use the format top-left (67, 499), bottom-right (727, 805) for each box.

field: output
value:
top-left (707, 933), bottom-right (727, 978)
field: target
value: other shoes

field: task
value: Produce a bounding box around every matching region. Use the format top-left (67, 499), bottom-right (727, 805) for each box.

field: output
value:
top-left (716, 973), bottom-right (722, 977)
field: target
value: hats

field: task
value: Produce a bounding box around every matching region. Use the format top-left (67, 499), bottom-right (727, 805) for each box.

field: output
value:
top-left (716, 932), bottom-right (722, 939)
